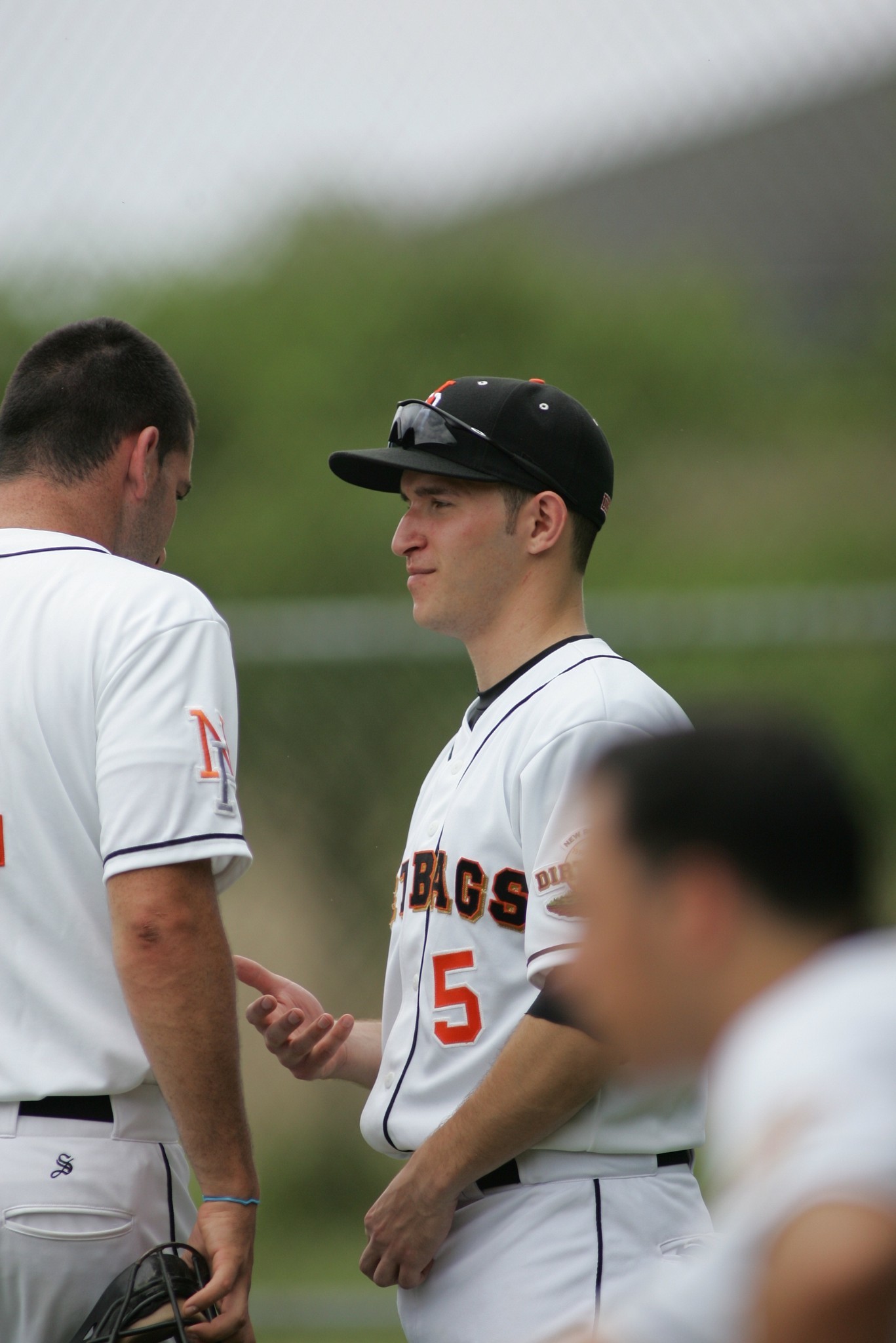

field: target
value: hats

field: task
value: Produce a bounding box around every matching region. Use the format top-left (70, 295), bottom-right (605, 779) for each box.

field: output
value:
top-left (327, 369), bottom-right (617, 529)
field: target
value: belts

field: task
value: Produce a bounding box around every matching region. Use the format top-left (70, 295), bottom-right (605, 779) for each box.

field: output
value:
top-left (19, 1093), bottom-right (114, 1122)
top-left (474, 1148), bottom-right (695, 1191)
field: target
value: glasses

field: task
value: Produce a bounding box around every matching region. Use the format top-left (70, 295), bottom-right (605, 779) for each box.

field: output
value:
top-left (388, 399), bottom-right (581, 508)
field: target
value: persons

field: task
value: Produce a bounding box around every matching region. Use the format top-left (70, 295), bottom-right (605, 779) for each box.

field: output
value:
top-left (0, 317), bottom-right (259, 1343)
top-left (547, 709), bottom-right (896, 1342)
top-left (231, 376), bottom-right (718, 1343)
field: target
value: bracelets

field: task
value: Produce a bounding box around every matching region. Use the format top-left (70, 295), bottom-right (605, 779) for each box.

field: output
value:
top-left (202, 1195), bottom-right (260, 1206)
